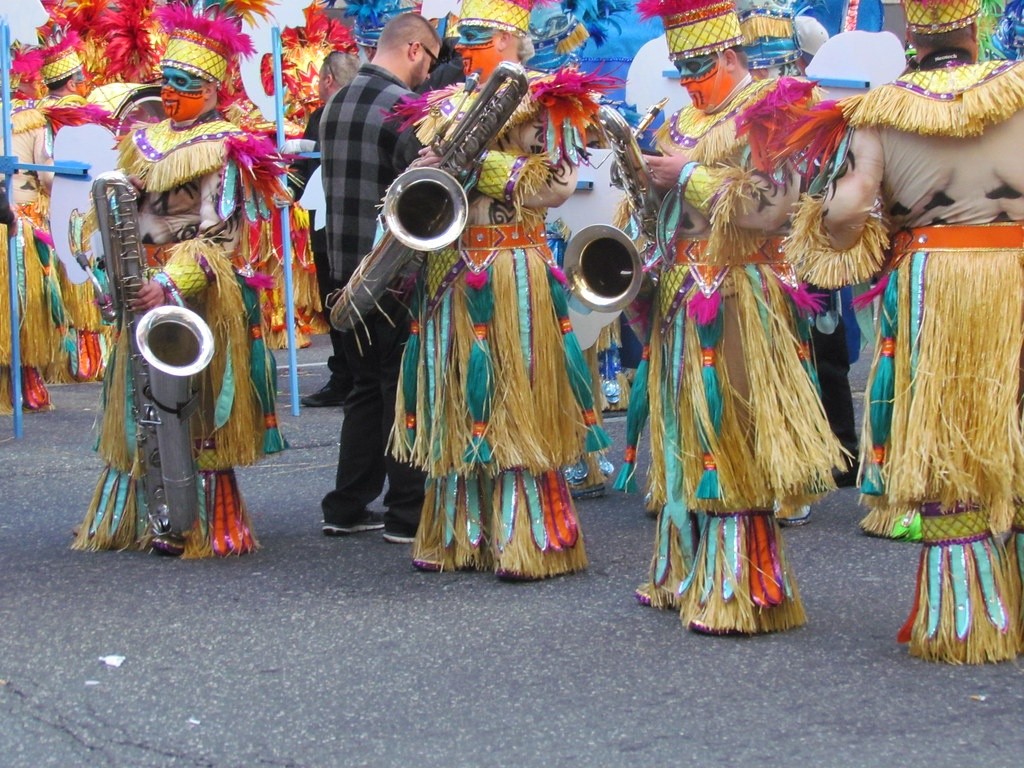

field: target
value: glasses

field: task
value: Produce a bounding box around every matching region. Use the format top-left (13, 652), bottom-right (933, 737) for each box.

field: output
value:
top-left (409, 41), bottom-right (440, 73)
top-left (328, 51), bottom-right (347, 79)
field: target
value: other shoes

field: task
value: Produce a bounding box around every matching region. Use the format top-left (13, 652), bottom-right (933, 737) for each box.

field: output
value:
top-left (383, 521), bottom-right (418, 544)
top-left (322, 511), bottom-right (385, 536)
top-left (828, 463), bottom-right (866, 487)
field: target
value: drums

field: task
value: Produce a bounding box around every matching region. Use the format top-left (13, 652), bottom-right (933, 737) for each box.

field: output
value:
top-left (49, 123), bottom-right (121, 284)
top-left (86, 80), bottom-right (171, 138)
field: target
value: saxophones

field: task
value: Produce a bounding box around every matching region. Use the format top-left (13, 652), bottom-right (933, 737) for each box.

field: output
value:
top-left (323, 60), bottom-right (530, 333)
top-left (74, 173), bottom-right (220, 542)
top-left (561, 96), bottom-right (672, 314)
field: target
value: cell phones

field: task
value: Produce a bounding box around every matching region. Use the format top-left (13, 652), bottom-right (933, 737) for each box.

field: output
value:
top-left (641, 149), bottom-right (664, 157)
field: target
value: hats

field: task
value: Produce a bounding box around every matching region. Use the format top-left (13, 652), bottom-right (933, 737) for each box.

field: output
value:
top-left (456, 1), bottom-right (560, 40)
top-left (902, 0), bottom-right (981, 34)
top-left (636, 0), bottom-right (745, 62)
top-left (37, 24), bottom-right (84, 85)
top-left (150, 0), bottom-right (258, 87)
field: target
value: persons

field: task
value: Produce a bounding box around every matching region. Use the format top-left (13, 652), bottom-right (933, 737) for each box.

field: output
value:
top-left (271, 49), bottom-right (360, 409)
top-left (819, 0), bottom-right (1024, 662)
top-left (316, 12), bottom-right (443, 542)
top-left (352, 0), bottom-right (924, 538)
top-left (73, 30), bottom-right (259, 556)
top-left (1, 41), bottom-right (58, 409)
top-left (636, 0), bottom-right (808, 637)
top-left (41, 42), bottom-right (107, 384)
top-left (411, 0), bottom-right (592, 579)
